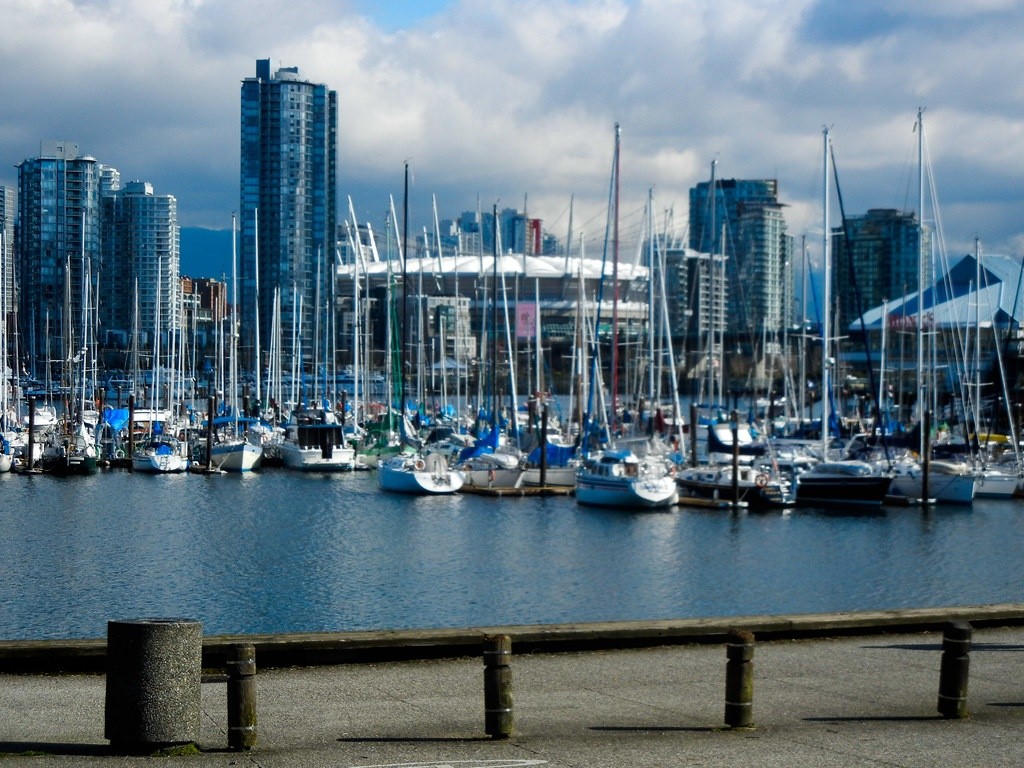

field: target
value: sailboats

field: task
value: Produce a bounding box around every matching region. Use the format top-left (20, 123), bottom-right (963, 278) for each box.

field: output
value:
top-left (0, 97), bottom-right (1024, 512)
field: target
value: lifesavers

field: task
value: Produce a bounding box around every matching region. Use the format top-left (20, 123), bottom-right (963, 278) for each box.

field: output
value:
top-left (54, 447), bottom-right (61, 457)
top-left (115, 450), bottom-right (125, 459)
top-left (488, 471), bottom-right (495, 482)
top-left (414, 458), bottom-right (426, 470)
top-left (754, 474), bottom-right (768, 487)
top-left (355, 454), bottom-right (368, 467)
